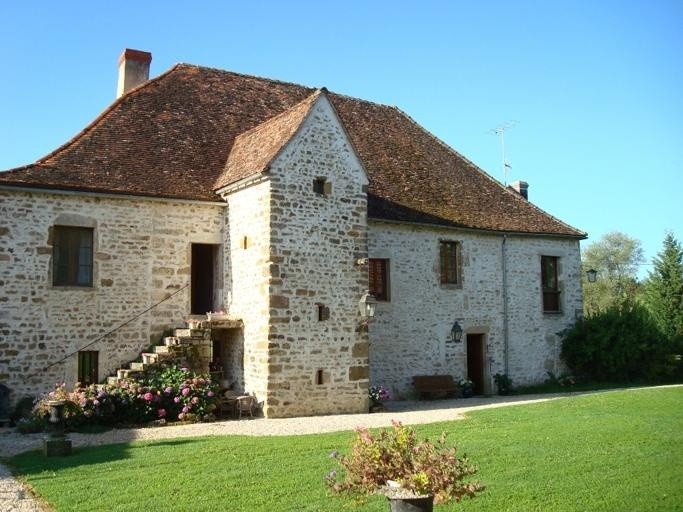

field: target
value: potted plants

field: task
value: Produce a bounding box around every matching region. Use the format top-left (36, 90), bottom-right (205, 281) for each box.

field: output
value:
top-left (493, 373), bottom-right (512, 396)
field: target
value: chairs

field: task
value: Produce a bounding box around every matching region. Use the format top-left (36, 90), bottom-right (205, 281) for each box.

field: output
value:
top-left (218, 390), bottom-right (255, 420)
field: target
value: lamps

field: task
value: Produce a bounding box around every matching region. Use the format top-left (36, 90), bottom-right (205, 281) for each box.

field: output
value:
top-left (451, 322), bottom-right (462, 343)
top-left (358, 289), bottom-right (377, 325)
top-left (586, 269), bottom-right (598, 283)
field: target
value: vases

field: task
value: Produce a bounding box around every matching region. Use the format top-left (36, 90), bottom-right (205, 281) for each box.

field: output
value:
top-left (373, 401), bottom-right (383, 407)
top-left (464, 388), bottom-right (473, 397)
top-left (387, 494), bottom-right (435, 512)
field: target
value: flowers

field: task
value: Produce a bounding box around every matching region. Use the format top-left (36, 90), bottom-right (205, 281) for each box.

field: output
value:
top-left (458, 378), bottom-right (474, 388)
top-left (325, 420), bottom-right (487, 506)
top-left (369, 384), bottom-right (391, 402)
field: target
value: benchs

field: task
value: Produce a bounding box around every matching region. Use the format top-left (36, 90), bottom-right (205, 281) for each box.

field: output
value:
top-left (413, 375), bottom-right (459, 400)
